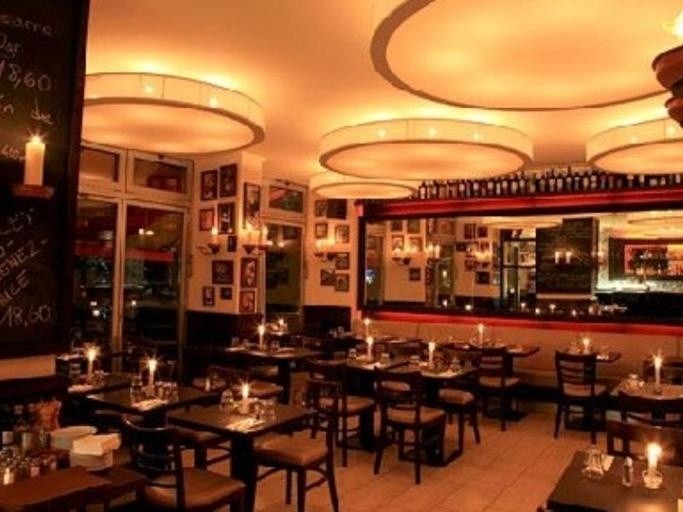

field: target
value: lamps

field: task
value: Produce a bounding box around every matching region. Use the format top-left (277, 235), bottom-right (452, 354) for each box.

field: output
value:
top-left (585, 115), bottom-right (682, 175)
top-left (80, 71), bottom-right (265, 162)
top-left (318, 118), bottom-right (534, 182)
top-left (369, 0), bottom-right (683, 111)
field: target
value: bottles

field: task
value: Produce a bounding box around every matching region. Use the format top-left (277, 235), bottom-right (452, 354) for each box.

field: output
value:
top-left (27, 404), bottom-right (37, 427)
top-left (0, 445), bottom-right (58, 485)
top-left (416, 165), bottom-right (682, 199)
top-left (14, 405), bottom-right (26, 433)
top-left (599, 343), bottom-right (611, 358)
top-left (623, 456), bottom-right (635, 487)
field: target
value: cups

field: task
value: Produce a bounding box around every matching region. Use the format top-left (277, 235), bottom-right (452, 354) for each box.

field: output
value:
top-left (130, 376), bottom-right (142, 395)
top-left (348, 348), bottom-right (356, 360)
top-left (381, 352), bottom-right (390, 364)
top-left (271, 340), bottom-right (278, 350)
top-left (643, 470), bottom-right (664, 489)
top-left (449, 359), bottom-right (460, 373)
top-left (262, 400), bottom-right (274, 418)
top-left (568, 342), bottom-right (580, 354)
top-left (230, 338), bottom-right (240, 348)
top-left (584, 444), bottom-right (601, 467)
top-left (408, 354), bottom-right (420, 367)
top-left (221, 389), bottom-right (232, 409)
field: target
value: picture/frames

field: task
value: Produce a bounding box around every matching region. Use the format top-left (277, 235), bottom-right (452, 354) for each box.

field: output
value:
top-left (198, 164), bottom-right (262, 315)
top-left (314, 199), bottom-right (350, 291)
top-left (388, 217), bottom-right (498, 286)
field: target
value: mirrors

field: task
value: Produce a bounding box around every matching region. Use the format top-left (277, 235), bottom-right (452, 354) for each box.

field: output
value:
top-left (352, 187), bottom-right (682, 337)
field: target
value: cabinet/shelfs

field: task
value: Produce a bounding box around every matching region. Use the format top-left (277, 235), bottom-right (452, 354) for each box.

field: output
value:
top-left (499, 237), bottom-right (536, 298)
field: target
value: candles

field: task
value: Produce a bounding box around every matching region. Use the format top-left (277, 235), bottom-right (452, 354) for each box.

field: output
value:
top-left (647, 454), bottom-right (657, 476)
top-left (655, 367), bottom-right (661, 385)
top-left (23, 140), bottom-right (46, 186)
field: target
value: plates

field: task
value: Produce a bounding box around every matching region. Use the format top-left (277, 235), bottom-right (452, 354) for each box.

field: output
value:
top-left (50, 425), bottom-right (93, 453)
top-left (68, 447), bottom-right (113, 473)
